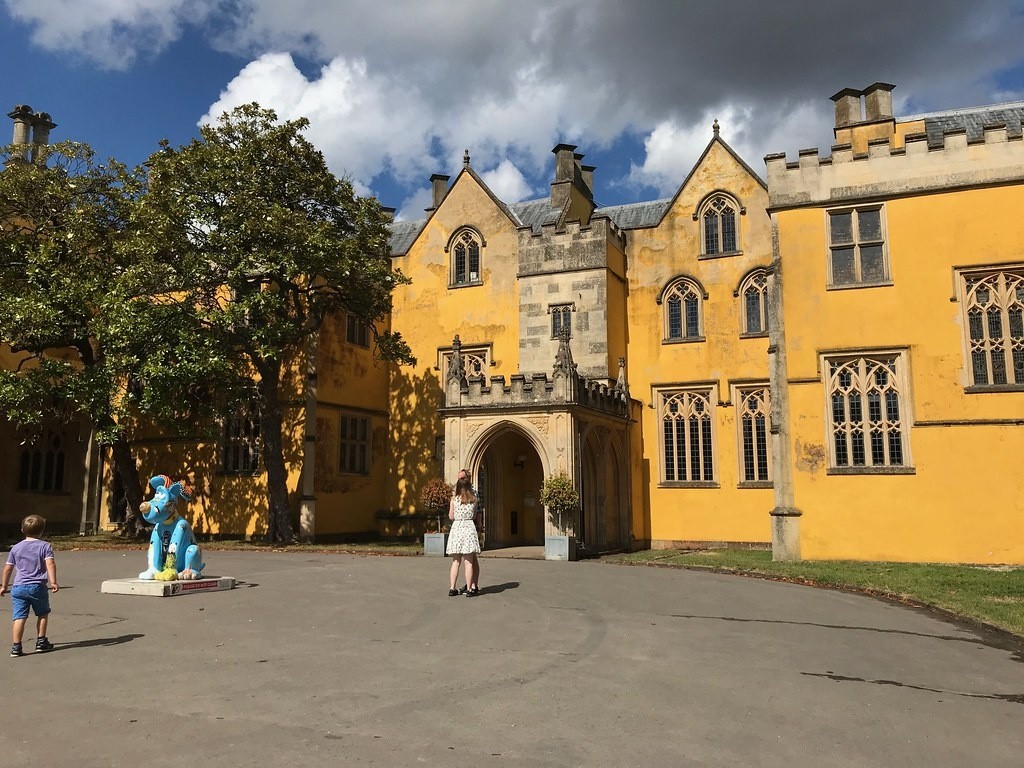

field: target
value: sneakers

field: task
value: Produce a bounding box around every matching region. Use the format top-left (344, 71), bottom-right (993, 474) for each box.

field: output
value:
top-left (35, 637), bottom-right (54, 651)
top-left (10, 646), bottom-right (23, 657)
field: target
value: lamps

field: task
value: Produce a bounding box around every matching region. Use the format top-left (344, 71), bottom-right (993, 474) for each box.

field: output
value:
top-left (514, 454), bottom-right (528, 470)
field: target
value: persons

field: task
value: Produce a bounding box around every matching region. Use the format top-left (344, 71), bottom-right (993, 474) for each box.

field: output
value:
top-left (0, 514), bottom-right (60, 657)
top-left (446, 468), bottom-right (483, 597)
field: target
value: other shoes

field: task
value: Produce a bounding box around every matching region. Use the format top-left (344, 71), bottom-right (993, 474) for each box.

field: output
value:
top-left (466, 589), bottom-right (478, 596)
top-left (449, 589), bottom-right (460, 596)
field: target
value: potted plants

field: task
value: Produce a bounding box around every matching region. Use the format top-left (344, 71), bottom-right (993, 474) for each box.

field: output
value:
top-left (420, 477), bottom-right (454, 558)
top-left (536, 466), bottom-right (581, 561)
